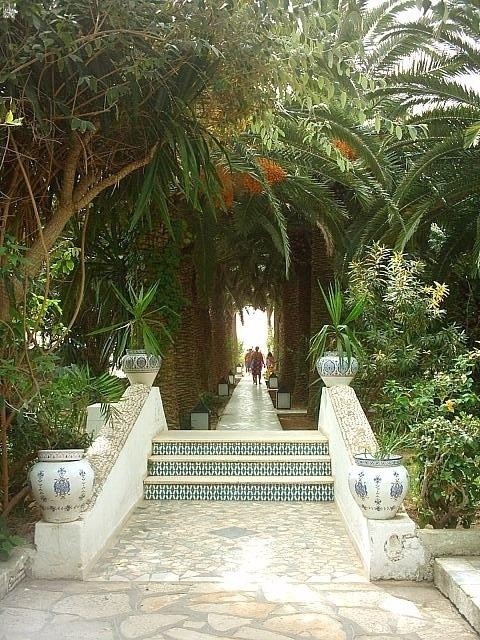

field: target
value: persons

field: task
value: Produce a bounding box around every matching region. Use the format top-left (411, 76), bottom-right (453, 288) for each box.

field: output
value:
top-left (245, 348), bottom-right (252, 373)
top-left (266, 352), bottom-right (274, 368)
top-left (251, 346), bottom-right (265, 385)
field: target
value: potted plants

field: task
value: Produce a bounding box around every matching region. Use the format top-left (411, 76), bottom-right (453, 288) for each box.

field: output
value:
top-left (84, 275), bottom-right (186, 387)
top-left (345, 416), bottom-right (412, 521)
top-left (302, 273), bottom-right (374, 387)
top-left (25, 395), bottom-right (97, 524)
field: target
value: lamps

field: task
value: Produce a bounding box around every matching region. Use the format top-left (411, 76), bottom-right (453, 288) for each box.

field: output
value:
top-left (269, 371), bottom-right (279, 390)
top-left (189, 397), bottom-right (209, 429)
top-left (276, 384), bottom-right (293, 410)
top-left (218, 363), bottom-right (243, 395)
